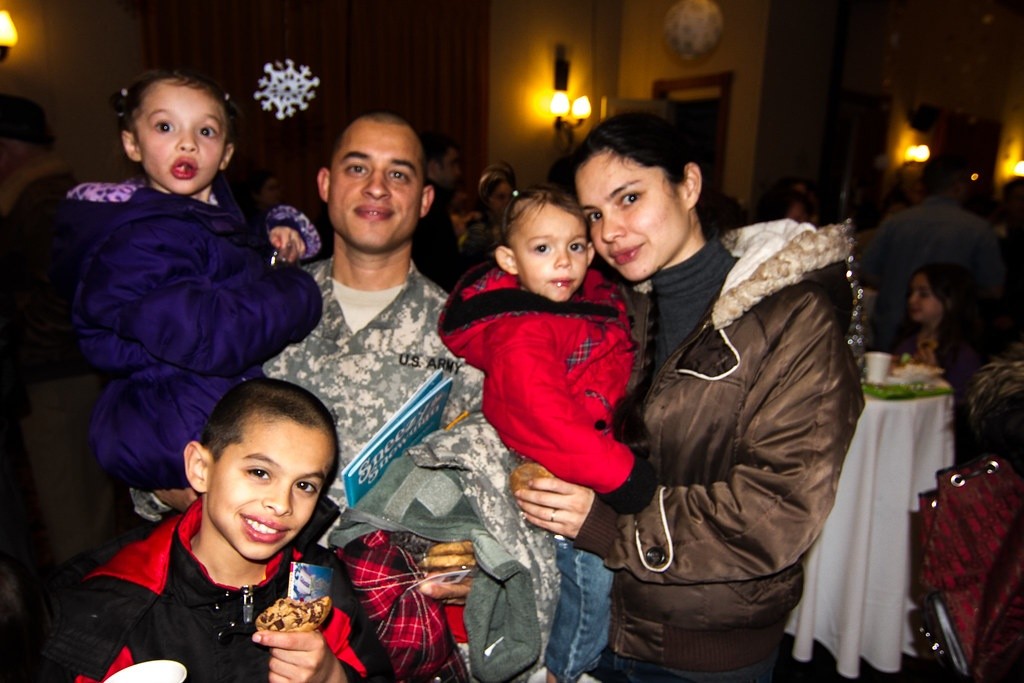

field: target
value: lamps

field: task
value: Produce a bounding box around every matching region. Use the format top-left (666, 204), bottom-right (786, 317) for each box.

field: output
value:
top-left (552, 92), bottom-right (591, 131)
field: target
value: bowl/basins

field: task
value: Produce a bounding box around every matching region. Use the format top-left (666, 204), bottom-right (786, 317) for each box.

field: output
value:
top-left (893, 365), bottom-right (944, 383)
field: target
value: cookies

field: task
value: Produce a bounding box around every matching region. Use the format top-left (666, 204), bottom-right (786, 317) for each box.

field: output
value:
top-left (256, 595), bottom-right (333, 632)
top-left (510, 463), bottom-right (555, 493)
top-left (420, 541), bottom-right (477, 569)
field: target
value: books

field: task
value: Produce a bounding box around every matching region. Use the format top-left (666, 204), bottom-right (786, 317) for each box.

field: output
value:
top-left (341, 368), bottom-right (452, 508)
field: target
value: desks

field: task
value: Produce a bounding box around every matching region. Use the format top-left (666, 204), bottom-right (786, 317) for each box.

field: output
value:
top-left (785, 378), bottom-right (955, 678)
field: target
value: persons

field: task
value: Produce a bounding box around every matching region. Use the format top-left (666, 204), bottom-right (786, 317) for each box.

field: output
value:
top-left (514, 109), bottom-right (864, 683)
top-left (0, 92), bottom-right (117, 683)
top-left (44, 381), bottom-right (396, 683)
top-left (438, 189), bottom-right (658, 683)
top-left (241, 169), bottom-right (277, 208)
top-left (756, 151), bottom-right (1024, 463)
top-left (409, 131), bottom-right (515, 293)
top-left (62, 68), bottom-right (322, 523)
top-left (155, 109), bottom-right (484, 604)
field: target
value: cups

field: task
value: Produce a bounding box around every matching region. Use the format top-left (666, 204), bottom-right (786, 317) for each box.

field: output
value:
top-left (101, 659), bottom-right (187, 683)
top-left (866, 353), bottom-right (891, 382)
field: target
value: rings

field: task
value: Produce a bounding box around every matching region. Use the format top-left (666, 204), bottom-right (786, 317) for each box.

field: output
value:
top-left (551, 509), bottom-right (556, 522)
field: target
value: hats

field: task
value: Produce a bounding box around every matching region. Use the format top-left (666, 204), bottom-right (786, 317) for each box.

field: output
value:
top-left (924, 260), bottom-right (977, 302)
top-left (1, 93), bottom-right (57, 149)
